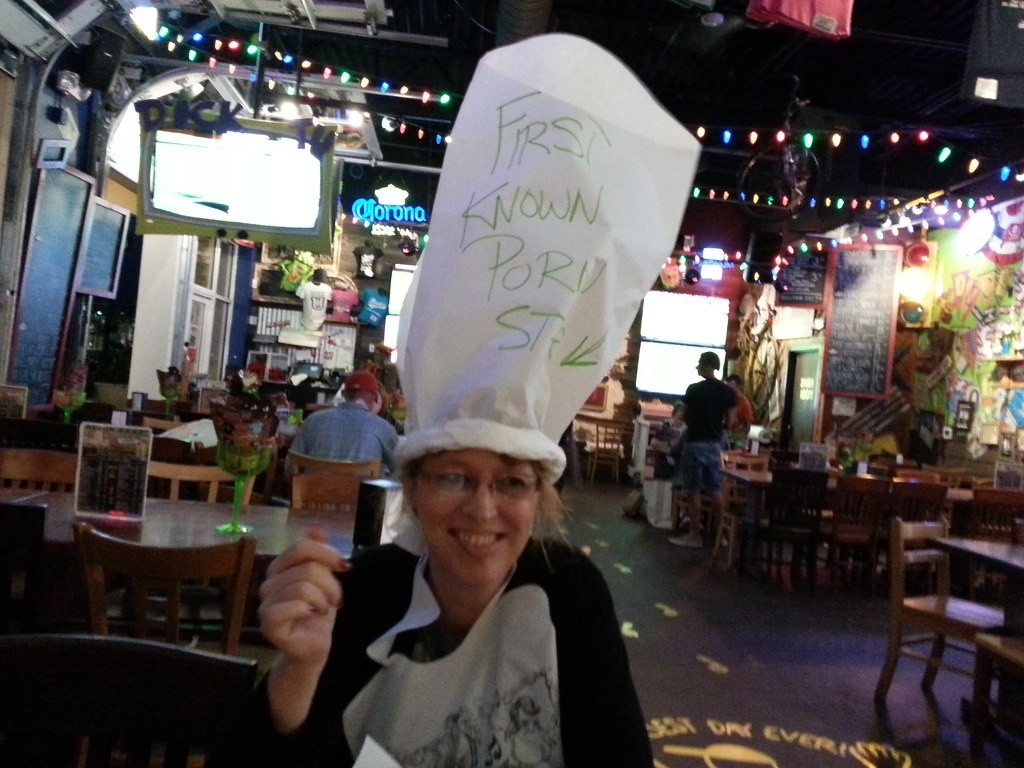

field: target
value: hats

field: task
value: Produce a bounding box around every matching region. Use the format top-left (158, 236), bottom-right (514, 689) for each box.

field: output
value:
top-left (391, 32), bottom-right (702, 485)
top-left (345, 370), bottom-right (378, 392)
top-left (702, 352), bottom-right (720, 371)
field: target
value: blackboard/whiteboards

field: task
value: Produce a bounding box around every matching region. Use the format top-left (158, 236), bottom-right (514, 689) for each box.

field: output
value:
top-left (6, 161), bottom-right (99, 422)
top-left (819, 242), bottom-right (904, 402)
top-left (76, 196), bottom-right (131, 301)
top-left (774, 232), bottom-right (836, 310)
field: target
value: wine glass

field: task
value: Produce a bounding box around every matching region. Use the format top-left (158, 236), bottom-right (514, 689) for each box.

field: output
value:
top-left (53, 391), bottom-right (88, 423)
top-left (156, 385), bottom-right (181, 413)
top-left (210, 444), bottom-right (280, 535)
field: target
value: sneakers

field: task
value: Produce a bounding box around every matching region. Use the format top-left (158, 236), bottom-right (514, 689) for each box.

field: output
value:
top-left (668, 534), bottom-right (703, 548)
top-left (713, 535), bottom-right (729, 547)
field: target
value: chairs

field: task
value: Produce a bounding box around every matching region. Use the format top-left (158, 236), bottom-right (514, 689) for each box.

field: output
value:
top-left (0, 446), bottom-right (78, 495)
top-left (0, 394), bottom-right (381, 513)
top-left (708, 445), bottom-right (1024, 598)
top-left (585, 421), bottom-right (626, 485)
top-left (0, 501), bottom-right (47, 639)
top-left (871, 516), bottom-right (1005, 706)
top-left (969, 633), bottom-right (1024, 761)
top-left (0, 632), bottom-right (258, 768)
top-left (70, 520), bottom-right (258, 662)
top-left (144, 458), bottom-right (256, 503)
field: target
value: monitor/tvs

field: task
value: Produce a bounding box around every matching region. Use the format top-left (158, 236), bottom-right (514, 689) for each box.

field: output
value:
top-left (136, 108), bottom-right (339, 255)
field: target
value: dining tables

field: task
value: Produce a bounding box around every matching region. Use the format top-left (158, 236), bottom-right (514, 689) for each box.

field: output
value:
top-left (0, 485), bottom-right (356, 648)
top-left (922, 535), bottom-right (1024, 729)
top-left (719, 467), bottom-right (975, 590)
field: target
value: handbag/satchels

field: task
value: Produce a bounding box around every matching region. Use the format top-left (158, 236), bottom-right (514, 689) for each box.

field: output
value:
top-left (643, 478), bottom-right (674, 529)
top-left (623, 488), bottom-right (643, 516)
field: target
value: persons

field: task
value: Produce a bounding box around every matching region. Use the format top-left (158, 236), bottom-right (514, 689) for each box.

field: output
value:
top-left (203, 381), bottom-right (653, 768)
top-left (636, 352), bottom-right (752, 547)
top-left (282, 368), bottom-right (404, 488)
